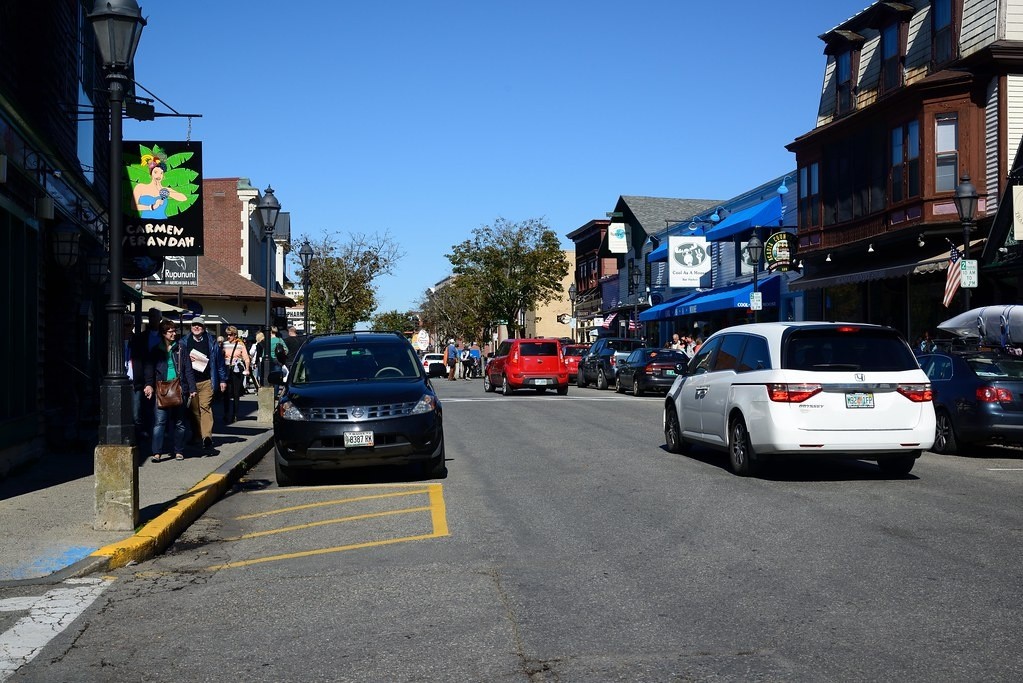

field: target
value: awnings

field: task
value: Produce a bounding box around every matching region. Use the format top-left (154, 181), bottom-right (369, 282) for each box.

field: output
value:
top-left (705, 196), bottom-right (782, 242)
top-left (639, 292), bottom-right (704, 322)
top-left (648, 226), bottom-right (703, 262)
top-left (675, 275), bottom-right (781, 317)
top-left (788, 238), bottom-right (984, 293)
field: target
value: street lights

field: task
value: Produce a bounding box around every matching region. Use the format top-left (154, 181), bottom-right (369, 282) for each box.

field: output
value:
top-left (299, 237), bottom-right (314, 336)
top-left (745, 231), bottom-right (764, 323)
top-left (83, 0), bottom-right (148, 528)
top-left (951, 168), bottom-right (980, 314)
top-left (254, 184), bottom-right (282, 386)
top-left (567, 282), bottom-right (578, 339)
top-left (631, 264), bottom-right (642, 338)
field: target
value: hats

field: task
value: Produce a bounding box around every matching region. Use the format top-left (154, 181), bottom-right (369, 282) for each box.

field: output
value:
top-left (270, 325), bottom-right (278, 332)
top-left (450, 339), bottom-right (455, 343)
top-left (191, 317), bottom-right (205, 326)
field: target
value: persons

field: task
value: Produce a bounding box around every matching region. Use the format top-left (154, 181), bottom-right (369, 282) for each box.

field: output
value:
top-left (473, 354), bottom-right (482, 377)
top-left (920, 330), bottom-right (938, 354)
top-left (460, 343), bottom-right (475, 381)
top-left (665, 325), bottom-right (713, 359)
top-left (124, 308), bottom-right (297, 463)
top-left (443, 339), bottom-right (459, 381)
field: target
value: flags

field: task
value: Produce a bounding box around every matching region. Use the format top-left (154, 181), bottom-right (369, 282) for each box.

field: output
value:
top-left (942, 245), bottom-right (966, 308)
top-left (629, 309), bottom-right (643, 331)
top-left (601, 313), bottom-right (617, 329)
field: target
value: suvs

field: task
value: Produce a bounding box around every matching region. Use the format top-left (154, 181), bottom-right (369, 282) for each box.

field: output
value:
top-left (577, 338), bottom-right (646, 390)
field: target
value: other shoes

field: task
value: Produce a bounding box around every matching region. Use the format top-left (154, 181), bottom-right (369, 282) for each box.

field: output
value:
top-left (151, 457), bottom-right (160, 462)
top-left (466, 377), bottom-right (472, 380)
top-left (204, 436), bottom-right (212, 451)
top-left (463, 378), bottom-right (465, 380)
top-left (176, 455), bottom-right (183, 460)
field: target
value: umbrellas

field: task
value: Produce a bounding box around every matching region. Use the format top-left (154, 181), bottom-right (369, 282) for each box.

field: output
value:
top-left (126, 299), bottom-right (188, 314)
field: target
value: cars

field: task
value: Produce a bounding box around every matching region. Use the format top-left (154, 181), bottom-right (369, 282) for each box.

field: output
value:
top-left (560, 343), bottom-right (592, 385)
top-left (661, 321), bottom-right (938, 476)
top-left (615, 347), bottom-right (690, 397)
top-left (268, 329), bottom-right (448, 486)
top-left (420, 353), bottom-right (451, 379)
top-left (484, 339), bottom-right (569, 395)
top-left (915, 348), bottom-right (1023, 455)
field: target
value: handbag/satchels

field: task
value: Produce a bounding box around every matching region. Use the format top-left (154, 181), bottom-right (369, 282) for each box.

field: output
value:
top-left (275, 338), bottom-right (286, 365)
top-left (226, 365), bottom-right (233, 378)
top-left (157, 379), bottom-right (183, 407)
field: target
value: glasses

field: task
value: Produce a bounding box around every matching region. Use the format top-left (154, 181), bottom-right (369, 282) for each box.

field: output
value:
top-left (228, 333), bottom-right (236, 336)
top-left (166, 330), bottom-right (176, 333)
top-left (191, 323), bottom-right (203, 327)
